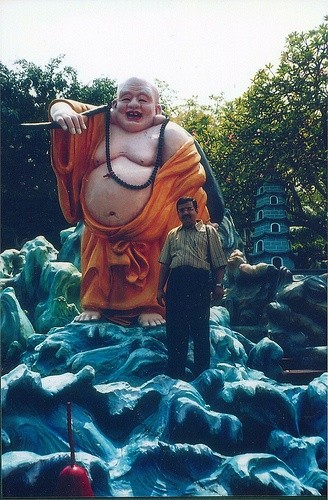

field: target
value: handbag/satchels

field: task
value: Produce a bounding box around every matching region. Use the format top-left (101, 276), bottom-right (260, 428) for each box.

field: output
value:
top-left (207, 278), bottom-right (217, 289)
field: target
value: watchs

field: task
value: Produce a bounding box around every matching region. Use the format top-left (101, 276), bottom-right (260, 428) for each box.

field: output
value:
top-left (215, 283), bottom-right (223, 289)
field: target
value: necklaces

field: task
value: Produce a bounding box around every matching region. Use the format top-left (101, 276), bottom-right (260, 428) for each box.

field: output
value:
top-left (105, 101), bottom-right (170, 190)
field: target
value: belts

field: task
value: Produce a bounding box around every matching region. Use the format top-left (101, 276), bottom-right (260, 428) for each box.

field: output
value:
top-left (172, 265), bottom-right (198, 273)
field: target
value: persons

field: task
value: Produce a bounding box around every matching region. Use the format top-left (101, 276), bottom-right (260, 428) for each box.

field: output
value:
top-left (156, 196), bottom-right (228, 379)
top-left (47, 77), bottom-right (219, 327)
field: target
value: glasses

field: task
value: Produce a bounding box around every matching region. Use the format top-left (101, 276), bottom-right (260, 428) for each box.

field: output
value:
top-left (177, 207), bottom-right (194, 212)
